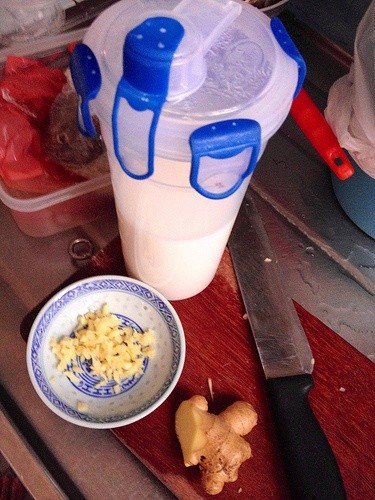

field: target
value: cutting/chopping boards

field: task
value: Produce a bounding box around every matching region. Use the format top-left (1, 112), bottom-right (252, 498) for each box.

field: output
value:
top-left (20, 236), bottom-right (375, 500)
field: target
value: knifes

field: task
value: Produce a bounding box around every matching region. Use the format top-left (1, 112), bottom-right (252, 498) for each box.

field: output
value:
top-left (226, 189), bottom-right (346, 500)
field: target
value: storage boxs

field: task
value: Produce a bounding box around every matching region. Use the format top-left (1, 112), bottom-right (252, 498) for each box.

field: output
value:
top-left (0, 26), bottom-right (115, 237)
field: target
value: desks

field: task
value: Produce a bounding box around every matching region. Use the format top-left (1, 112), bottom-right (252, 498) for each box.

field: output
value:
top-left (0, 82), bottom-right (375, 500)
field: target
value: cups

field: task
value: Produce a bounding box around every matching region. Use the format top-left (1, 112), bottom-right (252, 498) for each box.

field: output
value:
top-left (68, 0), bottom-right (305, 300)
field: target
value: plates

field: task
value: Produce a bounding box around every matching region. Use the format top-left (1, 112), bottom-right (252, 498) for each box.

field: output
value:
top-left (26, 275), bottom-right (187, 429)
